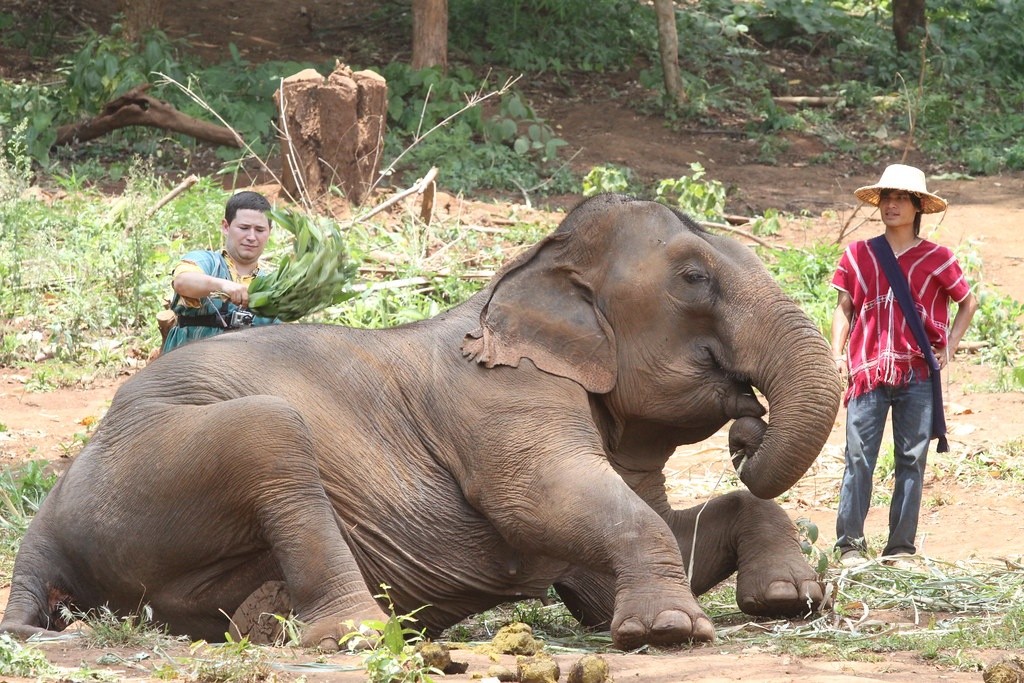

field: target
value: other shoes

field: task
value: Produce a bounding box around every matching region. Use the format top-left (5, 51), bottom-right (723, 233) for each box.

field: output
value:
top-left (884, 553), bottom-right (917, 568)
top-left (839, 549), bottom-right (868, 565)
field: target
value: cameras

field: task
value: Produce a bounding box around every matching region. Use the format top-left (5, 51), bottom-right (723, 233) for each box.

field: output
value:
top-left (230, 311), bottom-right (252, 328)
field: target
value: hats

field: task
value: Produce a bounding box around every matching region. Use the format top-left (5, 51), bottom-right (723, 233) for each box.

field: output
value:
top-left (853, 163), bottom-right (947, 214)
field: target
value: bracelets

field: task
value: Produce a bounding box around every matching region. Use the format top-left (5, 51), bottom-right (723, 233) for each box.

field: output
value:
top-left (834, 355), bottom-right (847, 361)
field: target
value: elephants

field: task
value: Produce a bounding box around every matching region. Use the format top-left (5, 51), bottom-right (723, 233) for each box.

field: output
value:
top-left (0, 193), bottom-right (840, 657)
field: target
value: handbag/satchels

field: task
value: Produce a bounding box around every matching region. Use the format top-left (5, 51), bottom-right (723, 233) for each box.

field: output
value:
top-left (929, 367), bottom-right (950, 454)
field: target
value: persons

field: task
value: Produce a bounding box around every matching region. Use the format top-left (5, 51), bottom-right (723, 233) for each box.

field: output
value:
top-left (831, 164), bottom-right (976, 570)
top-left (162, 191), bottom-right (281, 354)
